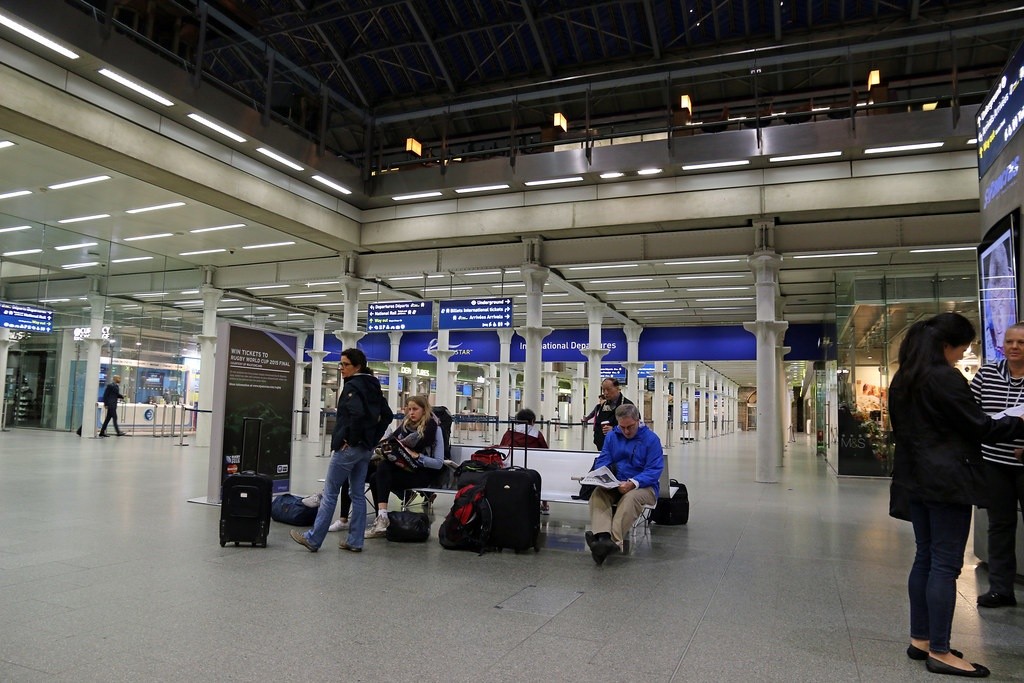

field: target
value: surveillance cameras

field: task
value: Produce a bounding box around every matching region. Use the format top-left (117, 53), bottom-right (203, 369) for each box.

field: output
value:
top-left (226, 248), bottom-right (235, 254)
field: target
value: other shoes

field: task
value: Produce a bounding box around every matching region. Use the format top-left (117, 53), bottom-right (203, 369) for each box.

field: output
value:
top-left (907, 643), bottom-right (963, 659)
top-left (585, 530), bottom-right (604, 566)
top-left (925, 655), bottom-right (990, 678)
top-left (99, 433), bottom-right (110, 437)
top-left (590, 538), bottom-right (621, 554)
top-left (117, 432), bottom-right (125, 436)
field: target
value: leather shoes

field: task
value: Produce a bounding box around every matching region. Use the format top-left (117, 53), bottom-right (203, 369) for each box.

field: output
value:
top-left (977, 589), bottom-right (1018, 607)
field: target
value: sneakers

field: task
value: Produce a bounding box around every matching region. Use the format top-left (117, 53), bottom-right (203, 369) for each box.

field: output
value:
top-left (364, 513), bottom-right (389, 539)
top-left (328, 519), bottom-right (350, 531)
top-left (290, 528), bottom-right (318, 552)
top-left (338, 539), bottom-right (362, 552)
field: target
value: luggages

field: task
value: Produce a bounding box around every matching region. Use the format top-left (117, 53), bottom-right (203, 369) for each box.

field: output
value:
top-left (479, 465), bottom-right (541, 554)
top-left (219, 417), bottom-right (273, 548)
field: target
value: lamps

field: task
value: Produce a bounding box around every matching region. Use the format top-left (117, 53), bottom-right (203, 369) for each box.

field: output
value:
top-left (681, 94), bottom-right (692, 116)
top-left (406, 137), bottom-right (422, 157)
top-left (867, 69), bottom-right (880, 92)
top-left (553, 112), bottom-right (567, 133)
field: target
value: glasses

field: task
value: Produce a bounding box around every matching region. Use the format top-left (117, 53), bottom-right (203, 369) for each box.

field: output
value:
top-left (338, 362), bottom-right (353, 369)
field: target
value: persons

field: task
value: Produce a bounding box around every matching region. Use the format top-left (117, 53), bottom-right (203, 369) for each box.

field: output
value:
top-left (585, 403), bottom-right (664, 564)
top-left (887, 313), bottom-right (1024, 677)
top-left (583, 377), bottom-right (641, 451)
top-left (98, 375), bottom-right (127, 437)
top-left (969, 322), bottom-right (1024, 606)
top-left (302, 396), bottom-right (444, 538)
top-left (500, 408), bottom-right (550, 514)
top-left (290, 348), bottom-right (394, 552)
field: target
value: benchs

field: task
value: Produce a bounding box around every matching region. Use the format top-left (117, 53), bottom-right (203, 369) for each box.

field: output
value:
top-left (402, 445), bottom-right (679, 536)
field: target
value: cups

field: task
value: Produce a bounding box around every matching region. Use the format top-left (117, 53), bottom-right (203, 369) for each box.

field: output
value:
top-left (601, 421), bottom-right (610, 435)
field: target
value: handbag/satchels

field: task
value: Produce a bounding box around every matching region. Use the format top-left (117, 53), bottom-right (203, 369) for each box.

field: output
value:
top-left (272, 493), bottom-right (321, 526)
top-left (651, 478), bottom-right (689, 526)
top-left (571, 457), bottom-right (599, 501)
top-left (438, 448), bottom-right (504, 552)
top-left (385, 510), bottom-right (429, 543)
